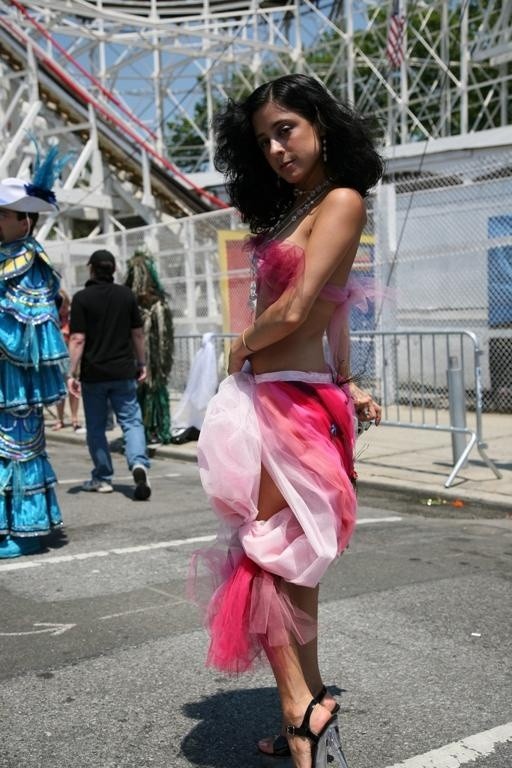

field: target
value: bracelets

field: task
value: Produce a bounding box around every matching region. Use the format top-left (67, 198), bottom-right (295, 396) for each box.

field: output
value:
top-left (64, 371), bottom-right (75, 382)
top-left (137, 362), bottom-right (147, 368)
top-left (242, 328), bottom-right (257, 354)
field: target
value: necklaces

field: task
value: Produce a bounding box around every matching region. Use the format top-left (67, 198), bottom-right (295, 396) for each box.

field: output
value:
top-left (261, 175), bottom-right (341, 245)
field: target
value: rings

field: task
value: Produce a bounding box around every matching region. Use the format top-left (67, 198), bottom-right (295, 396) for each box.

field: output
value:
top-left (364, 411), bottom-right (368, 416)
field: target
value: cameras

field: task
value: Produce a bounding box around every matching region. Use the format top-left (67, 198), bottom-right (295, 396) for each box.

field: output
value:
top-left (358, 412), bottom-right (372, 436)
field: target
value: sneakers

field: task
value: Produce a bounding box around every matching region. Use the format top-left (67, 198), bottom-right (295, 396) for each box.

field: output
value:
top-left (81, 477), bottom-right (113, 494)
top-left (49, 420), bottom-right (83, 431)
top-left (131, 462), bottom-right (152, 501)
top-left (0, 534), bottom-right (43, 560)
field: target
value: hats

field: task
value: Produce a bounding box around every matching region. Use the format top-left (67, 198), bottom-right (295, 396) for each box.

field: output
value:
top-left (86, 249), bottom-right (115, 267)
top-left (0, 176), bottom-right (57, 214)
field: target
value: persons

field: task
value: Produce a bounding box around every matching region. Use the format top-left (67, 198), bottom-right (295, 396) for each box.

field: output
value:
top-left (65, 248), bottom-right (154, 502)
top-left (195, 76), bottom-right (382, 768)
top-left (117, 248), bottom-right (174, 447)
top-left (0, 177), bottom-right (65, 563)
top-left (48, 290), bottom-right (82, 433)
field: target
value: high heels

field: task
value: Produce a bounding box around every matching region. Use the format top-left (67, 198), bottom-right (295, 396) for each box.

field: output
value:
top-left (282, 698), bottom-right (349, 768)
top-left (255, 683), bottom-right (343, 760)
